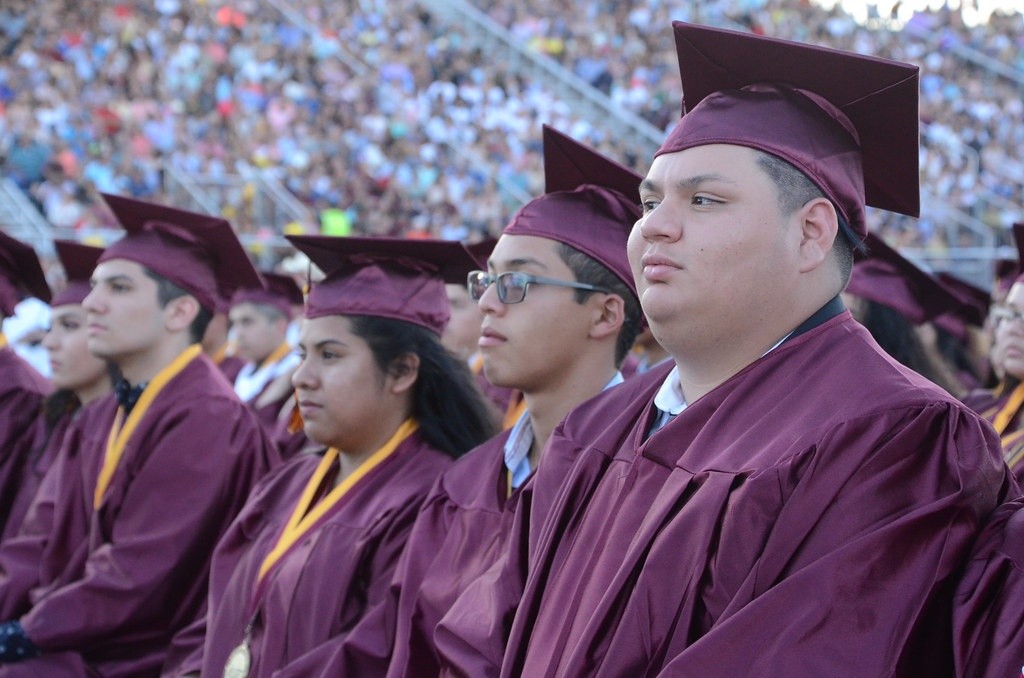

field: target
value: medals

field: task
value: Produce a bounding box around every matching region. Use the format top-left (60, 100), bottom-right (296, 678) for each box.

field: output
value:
top-left (220, 636), bottom-right (254, 678)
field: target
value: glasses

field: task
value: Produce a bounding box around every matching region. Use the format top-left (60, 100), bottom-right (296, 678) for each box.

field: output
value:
top-left (466, 271), bottom-right (607, 304)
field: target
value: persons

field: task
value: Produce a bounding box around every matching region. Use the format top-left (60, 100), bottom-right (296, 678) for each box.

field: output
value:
top-left (0, 0), bottom-right (1024, 678)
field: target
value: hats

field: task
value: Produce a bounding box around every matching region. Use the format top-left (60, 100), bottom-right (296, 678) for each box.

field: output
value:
top-left (503, 123), bottom-right (657, 292)
top-left (285, 234), bottom-right (481, 334)
top-left (51, 240), bottom-right (104, 308)
top-left (653, 22), bottom-right (921, 239)
top-left (0, 232), bottom-right (52, 314)
top-left (235, 272), bottom-right (302, 322)
top-left (846, 231), bottom-right (957, 325)
top-left (93, 193), bottom-right (266, 309)
top-left (932, 221), bottom-right (1024, 334)
